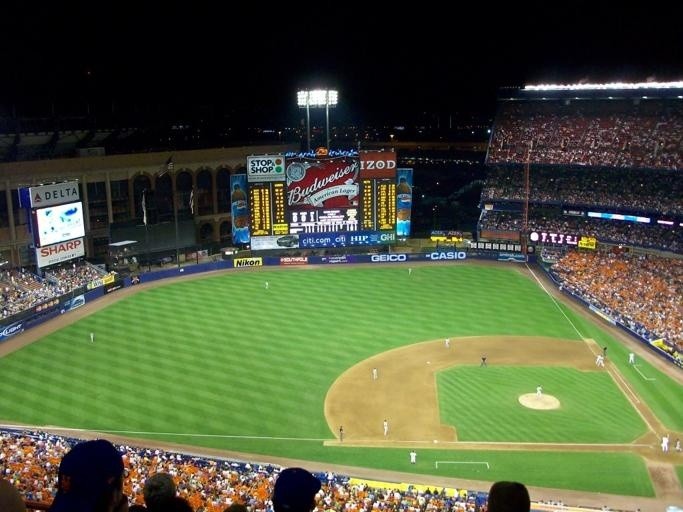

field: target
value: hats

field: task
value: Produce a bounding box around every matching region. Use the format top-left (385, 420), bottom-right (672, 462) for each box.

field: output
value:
top-left (143, 473), bottom-right (175, 507)
top-left (49, 439), bottom-right (123, 512)
top-left (275, 468), bottom-right (321, 507)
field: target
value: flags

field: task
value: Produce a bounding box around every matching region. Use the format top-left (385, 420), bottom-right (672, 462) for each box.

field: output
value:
top-left (157, 154), bottom-right (176, 178)
top-left (189, 189), bottom-right (194, 214)
top-left (142, 192), bottom-right (148, 226)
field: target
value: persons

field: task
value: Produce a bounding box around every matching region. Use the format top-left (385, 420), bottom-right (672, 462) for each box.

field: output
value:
top-left (1, 432), bottom-right (565, 511)
top-left (445, 338), bottom-right (450, 347)
top-left (340, 426), bottom-right (344, 441)
top-left (383, 419), bottom-right (388, 436)
top-left (0, 263), bottom-right (104, 320)
top-left (629, 353), bottom-right (635, 364)
top-left (661, 436), bottom-right (669, 452)
top-left (480, 355), bottom-right (487, 368)
top-left (408, 267), bottom-right (412, 275)
top-left (675, 438), bottom-right (683, 453)
top-left (596, 355), bottom-right (605, 368)
top-left (536, 384), bottom-right (542, 400)
top-left (265, 281), bottom-right (268, 289)
top-left (482, 100), bottom-right (683, 352)
top-left (90, 332), bottom-right (93, 342)
top-left (372, 367), bottom-right (377, 380)
